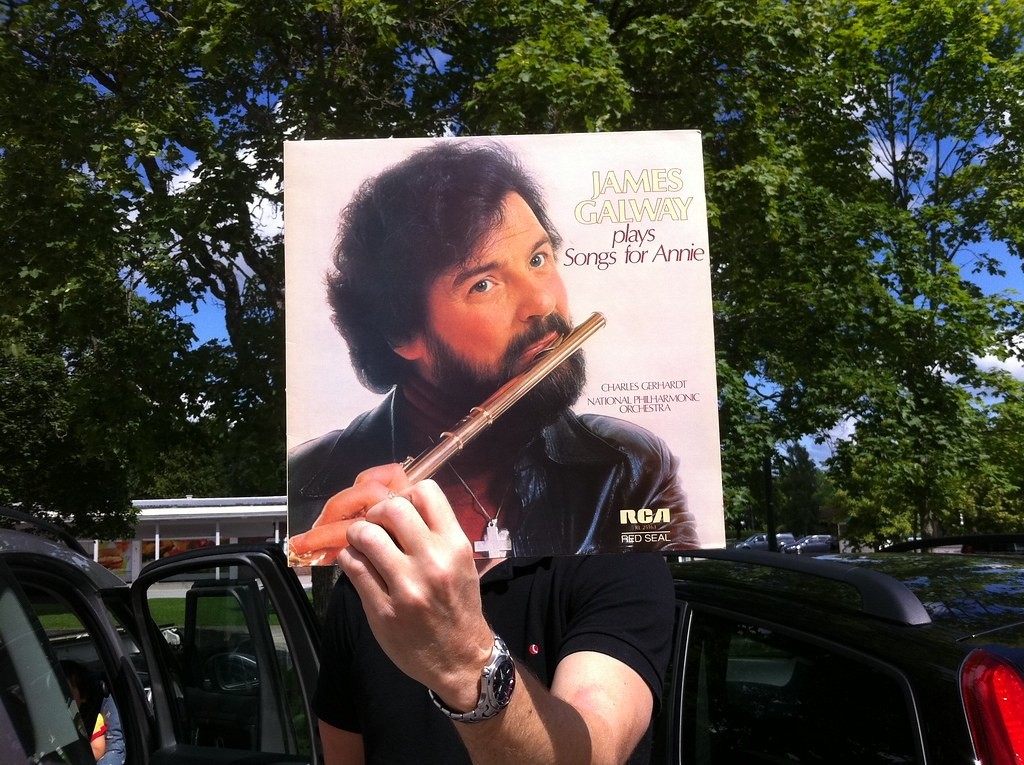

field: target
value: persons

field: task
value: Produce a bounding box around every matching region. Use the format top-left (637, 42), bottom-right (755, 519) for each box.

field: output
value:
top-left (314, 479), bottom-right (677, 765)
top-left (61, 659), bottom-right (108, 761)
top-left (287, 141), bottom-right (702, 567)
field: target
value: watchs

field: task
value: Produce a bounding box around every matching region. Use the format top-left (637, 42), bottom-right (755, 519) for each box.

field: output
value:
top-left (428, 633), bottom-right (516, 724)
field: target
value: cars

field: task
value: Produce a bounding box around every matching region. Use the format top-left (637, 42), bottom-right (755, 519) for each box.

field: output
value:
top-left (734, 533), bottom-right (795, 553)
top-left (783, 535), bottom-right (831, 555)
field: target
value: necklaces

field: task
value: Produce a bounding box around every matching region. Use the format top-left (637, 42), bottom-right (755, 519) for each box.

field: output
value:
top-left (428, 436), bottom-right (513, 558)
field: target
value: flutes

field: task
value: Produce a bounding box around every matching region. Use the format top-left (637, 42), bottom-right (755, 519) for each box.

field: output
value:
top-left (291, 310), bottom-right (607, 566)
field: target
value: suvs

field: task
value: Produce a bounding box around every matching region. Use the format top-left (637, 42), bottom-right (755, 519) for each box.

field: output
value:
top-left (0, 506), bottom-right (327, 765)
top-left (650, 533), bottom-right (1024, 765)
